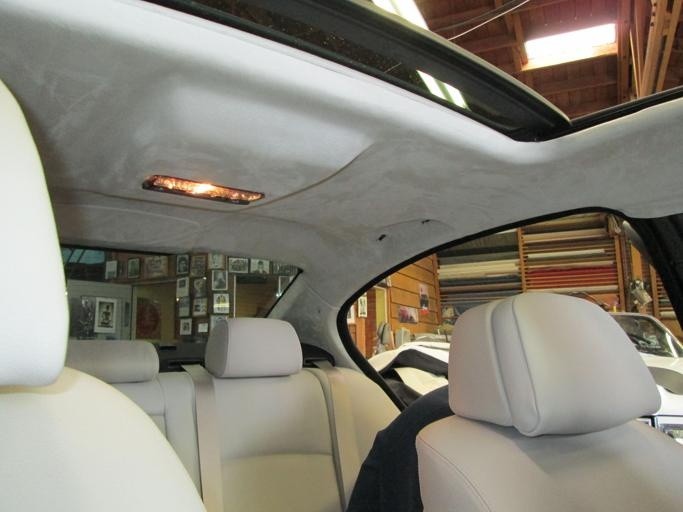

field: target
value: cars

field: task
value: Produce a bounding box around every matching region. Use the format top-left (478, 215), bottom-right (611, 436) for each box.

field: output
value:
top-left (606, 311), bottom-right (682, 446)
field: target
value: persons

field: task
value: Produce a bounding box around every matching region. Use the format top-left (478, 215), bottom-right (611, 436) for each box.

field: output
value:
top-left (252, 259), bottom-right (268, 275)
top-left (193, 278), bottom-right (206, 297)
top-left (217, 294), bottom-right (226, 311)
top-left (128, 260), bottom-right (138, 275)
top-left (90, 299), bottom-right (95, 314)
top-left (360, 299), bottom-right (366, 316)
top-left (99, 304), bottom-right (112, 327)
top-left (236, 259), bottom-right (247, 271)
top-left (147, 255), bottom-right (164, 277)
top-left (210, 254), bottom-right (223, 269)
top-left (213, 270), bottom-right (225, 288)
top-left (81, 300), bottom-right (90, 316)
top-left (177, 256), bottom-right (188, 273)
top-left (419, 286), bottom-right (429, 310)
